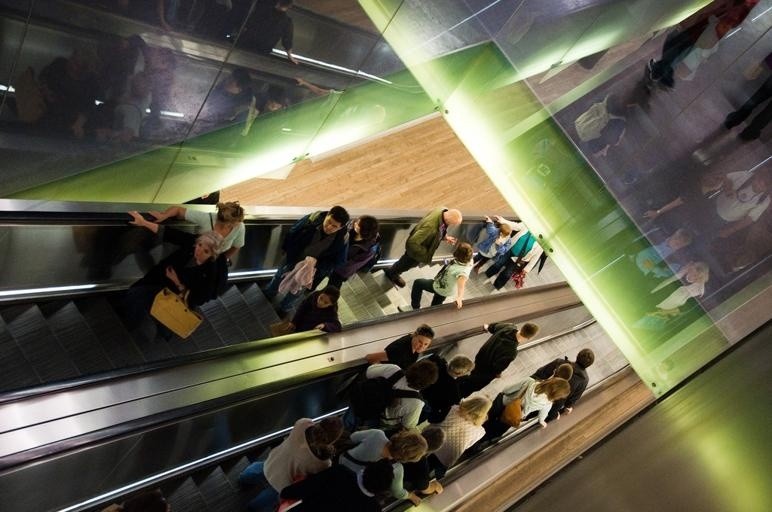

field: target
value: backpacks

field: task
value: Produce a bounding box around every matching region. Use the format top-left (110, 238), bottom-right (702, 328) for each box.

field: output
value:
top-left (355, 243), bottom-right (384, 273)
top-left (349, 368), bottom-right (419, 419)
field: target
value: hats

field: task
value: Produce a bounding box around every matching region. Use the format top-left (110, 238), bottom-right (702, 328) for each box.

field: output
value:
top-left (194, 230), bottom-right (224, 255)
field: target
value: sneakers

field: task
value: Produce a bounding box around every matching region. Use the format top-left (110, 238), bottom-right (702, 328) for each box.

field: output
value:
top-left (385, 269), bottom-right (407, 288)
top-left (396, 303), bottom-right (415, 312)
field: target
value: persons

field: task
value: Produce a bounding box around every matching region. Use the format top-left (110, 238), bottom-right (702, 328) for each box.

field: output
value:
top-left (241, 322), bottom-right (593, 512)
top-left (107, 489), bottom-right (169, 512)
top-left (1, 1), bottom-right (348, 144)
top-left (102, 200), bottom-right (554, 334)
top-left (562, 17), bottom-right (771, 330)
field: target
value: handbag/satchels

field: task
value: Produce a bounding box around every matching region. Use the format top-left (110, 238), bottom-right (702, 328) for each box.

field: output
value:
top-left (502, 397), bottom-right (523, 428)
top-left (149, 283), bottom-right (205, 340)
